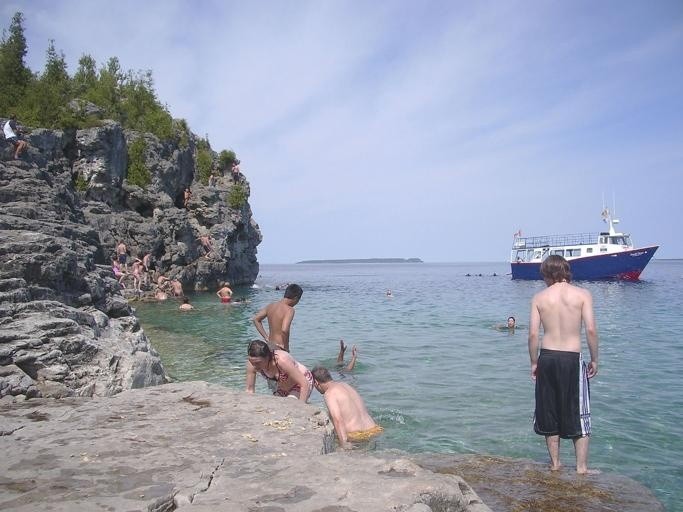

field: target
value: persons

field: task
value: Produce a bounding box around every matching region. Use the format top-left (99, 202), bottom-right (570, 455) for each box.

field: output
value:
top-left (112, 239), bottom-right (183, 302)
top-left (195, 235), bottom-right (215, 259)
top-left (241, 297), bottom-right (251, 303)
top-left (230, 297), bottom-right (242, 304)
top-left (252, 283), bottom-right (303, 352)
top-left (310, 367), bottom-right (383, 452)
top-left (526, 255), bottom-right (602, 475)
top-left (337, 339), bottom-right (357, 370)
top-left (179, 296), bottom-right (193, 311)
top-left (246, 340), bottom-right (314, 404)
top-left (495, 317), bottom-right (520, 328)
top-left (215, 282), bottom-right (233, 302)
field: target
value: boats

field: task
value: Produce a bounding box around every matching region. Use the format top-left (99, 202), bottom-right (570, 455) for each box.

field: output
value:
top-left (505, 199), bottom-right (660, 283)
